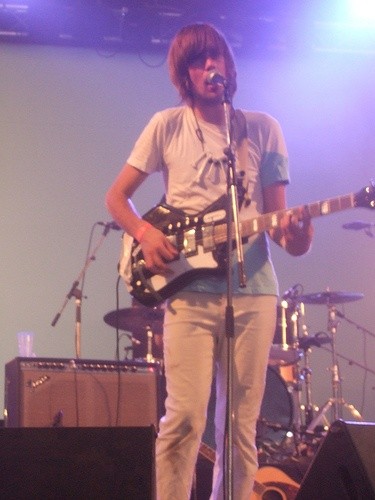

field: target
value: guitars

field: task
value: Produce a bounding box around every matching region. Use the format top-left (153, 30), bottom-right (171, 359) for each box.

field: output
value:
top-left (114, 180), bottom-right (375, 307)
top-left (199, 441), bottom-right (301, 500)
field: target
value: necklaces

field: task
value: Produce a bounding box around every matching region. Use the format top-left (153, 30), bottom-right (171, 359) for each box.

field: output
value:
top-left (186, 101), bottom-right (241, 184)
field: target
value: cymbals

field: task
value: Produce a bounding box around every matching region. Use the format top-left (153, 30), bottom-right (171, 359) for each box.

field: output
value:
top-left (101, 304), bottom-right (165, 335)
top-left (297, 287), bottom-right (366, 308)
top-left (296, 335), bottom-right (333, 350)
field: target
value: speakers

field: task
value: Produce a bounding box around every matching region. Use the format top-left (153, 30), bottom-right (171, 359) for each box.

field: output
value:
top-left (5, 357), bottom-right (159, 426)
top-left (290, 420), bottom-right (375, 500)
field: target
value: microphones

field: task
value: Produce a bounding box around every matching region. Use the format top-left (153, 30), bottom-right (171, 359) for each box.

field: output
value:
top-left (207, 73), bottom-right (227, 84)
top-left (277, 284), bottom-right (296, 305)
top-left (342, 222), bottom-right (372, 230)
top-left (99, 220), bottom-right (121, 230)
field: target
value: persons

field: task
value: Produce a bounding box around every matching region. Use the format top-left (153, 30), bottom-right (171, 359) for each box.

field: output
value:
top-left (102, 21), bottom-right (313, 500)
top-left (131, 330), bottom-right (164, 360)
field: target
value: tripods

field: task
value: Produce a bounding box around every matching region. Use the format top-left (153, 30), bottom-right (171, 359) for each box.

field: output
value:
top-left (298, 309), bottom-right (362, 453)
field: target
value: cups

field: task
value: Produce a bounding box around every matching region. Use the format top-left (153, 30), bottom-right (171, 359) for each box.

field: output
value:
top-left (16, 331), bottom-right (34, 356)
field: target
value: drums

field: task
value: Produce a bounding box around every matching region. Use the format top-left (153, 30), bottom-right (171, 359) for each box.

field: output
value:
top-left (197, 366), bottom-right (302, 458)
top-left (270, 303), bottom-right (302, 363)
top-left (131, 332), bottom-right (165, 372)
